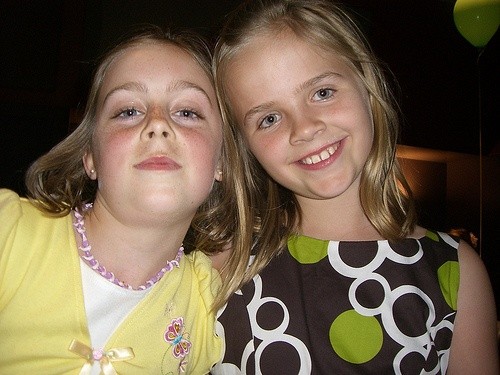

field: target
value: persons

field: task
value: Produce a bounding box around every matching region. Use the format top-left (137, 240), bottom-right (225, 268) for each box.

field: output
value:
top-left (209, 0), bottom-right (500, 375)
top-left (0, 24), bottom-right (237, 375)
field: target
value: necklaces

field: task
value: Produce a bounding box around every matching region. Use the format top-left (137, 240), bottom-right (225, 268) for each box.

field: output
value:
top-left (74, 204), bottom-right (184, 291)
